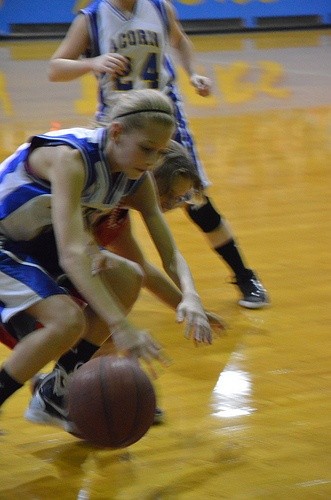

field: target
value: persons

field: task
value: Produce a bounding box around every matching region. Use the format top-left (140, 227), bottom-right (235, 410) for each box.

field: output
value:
top-left (0, 90), bottom-right (212, 436)
top-left (0, 142), bottom-right (227, 424)
top-left (47, 1), bottom-right (269, 308)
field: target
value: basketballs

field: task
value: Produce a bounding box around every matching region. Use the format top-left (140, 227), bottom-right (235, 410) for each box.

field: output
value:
top-left (64, 354), bottom-right (153, 448)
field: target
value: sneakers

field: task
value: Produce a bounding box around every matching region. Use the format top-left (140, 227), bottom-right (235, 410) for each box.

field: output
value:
top-left (238, 277), bottom-right (270, 309)
top-left (25, 369), bottom-right (163, 439)
